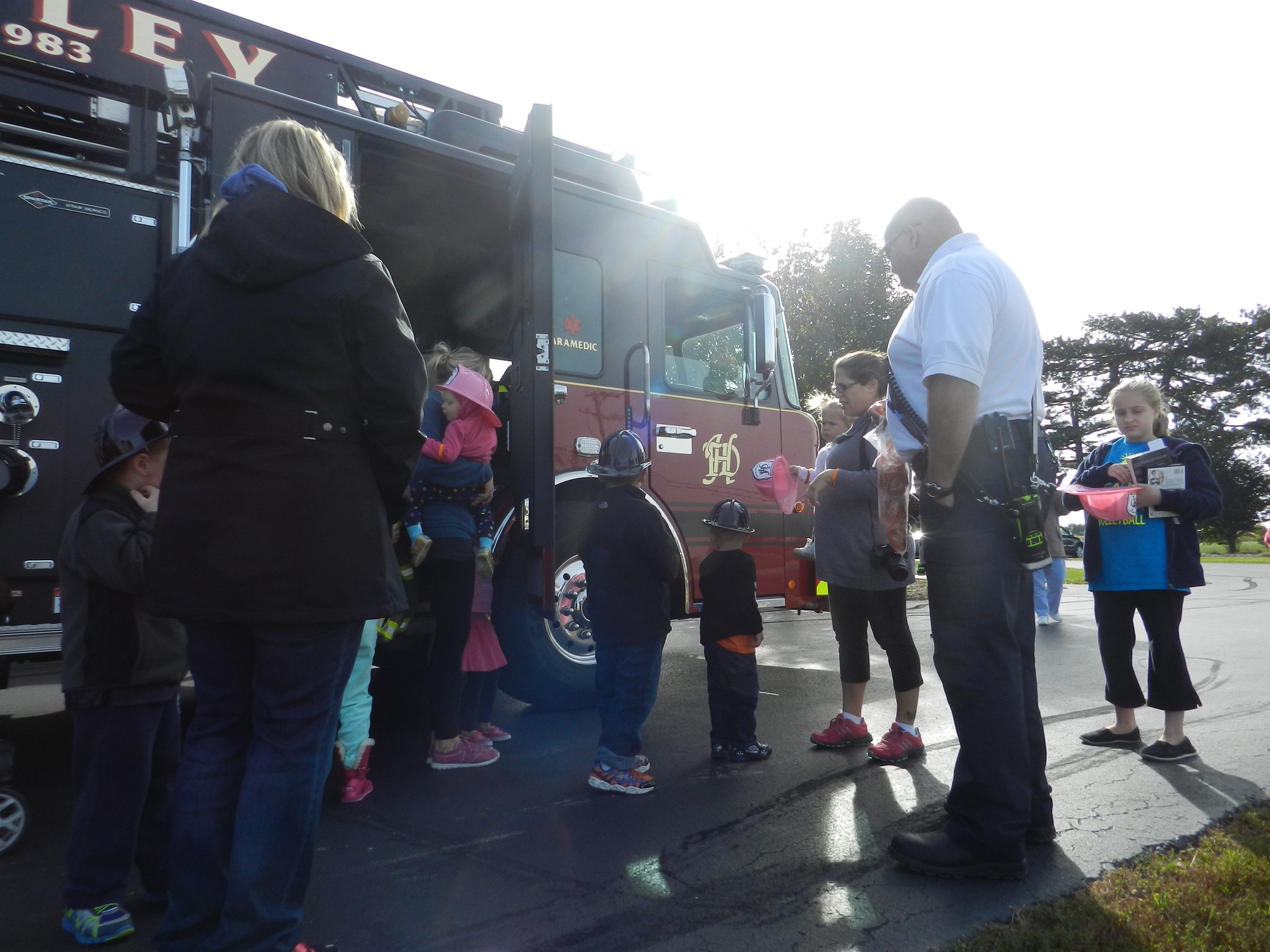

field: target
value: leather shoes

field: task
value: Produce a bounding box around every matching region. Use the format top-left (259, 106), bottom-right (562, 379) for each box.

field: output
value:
top-left (888, 817), bottom-right (1028, 880)
top-left (1028, 803), bottom-right (1056, 843)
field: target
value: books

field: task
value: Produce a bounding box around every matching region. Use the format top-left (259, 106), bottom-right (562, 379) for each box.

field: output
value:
top-left (1142, 463), bottom-right (1186, 518)
top-left (1126, 446), bottom-right (1172, 511)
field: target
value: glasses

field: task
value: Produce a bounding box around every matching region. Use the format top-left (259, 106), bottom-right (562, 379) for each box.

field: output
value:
top-left (830, 381), bottom-right (858, 394)
top-left (913, 484), bottom-right (921, 488)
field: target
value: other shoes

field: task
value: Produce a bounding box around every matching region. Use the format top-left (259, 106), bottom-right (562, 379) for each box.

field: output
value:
top-left (335, 738), bottom-right (374, 803)
top-left (476, 547), bottom-right (494, 580)
top-left (410, 534), bottom-right (433, 567)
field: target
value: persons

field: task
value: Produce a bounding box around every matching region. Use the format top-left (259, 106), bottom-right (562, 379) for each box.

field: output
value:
top-left (415, 343), bottom-right (501, 767)
top-left (579, 429), bottom-right (681, 795)
top-left (697, 497), bottom-right (773, 763)
top-left (109, 118), bottom-right (421, 952)
top-left (806, 348), bottom-right (926, 763)
top-left (1059, 373), bottom-right (1227, 761)
top-left (882, 196), bottom-right (1059, 882)
top-left (457, 554), bottom-right (513, 748)
top-left (1149, 470), bottom-right (1160, 485)
top-left (333, 618), bottom-right (381, 804)
top-left (1031, 489), bottom-right (1072, 626)
top-left (788, 390), bottom-right (858, 561)
top-left (400, 365), bottom-right (497, 580)
top-left (51, 401), bottom-right (186, 943)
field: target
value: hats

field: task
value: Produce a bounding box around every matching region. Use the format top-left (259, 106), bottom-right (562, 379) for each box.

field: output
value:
top-left (1056, 484), bottom-right (1144, 521)
top-left (87, 407), bottom-right (171, 490)
top-left (750, 454), bottom-right (798, 515)
top-left (701, 498), bottom-right (755, 533)
top-left (435, 363), bottom-right (502, 427)
top-left (585, 430), bottom-right (651, 477)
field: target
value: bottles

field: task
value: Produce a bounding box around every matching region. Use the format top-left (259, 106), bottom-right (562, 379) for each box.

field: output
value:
top-left (1009, 483), bottom-right (1053, 574)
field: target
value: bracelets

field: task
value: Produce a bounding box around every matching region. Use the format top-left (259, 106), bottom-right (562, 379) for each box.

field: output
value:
top-left (803, 469), bottom-right (810, 484)
top-left (832, 468), bottom-right (840, 487)
top-left (438, 443), bottom-right (444, 462)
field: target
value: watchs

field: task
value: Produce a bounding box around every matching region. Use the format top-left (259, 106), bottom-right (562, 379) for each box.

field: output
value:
top-left (921, 479), bottom-right (955, 501)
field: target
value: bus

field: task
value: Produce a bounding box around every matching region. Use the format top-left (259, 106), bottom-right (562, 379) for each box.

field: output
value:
top-left (0, 1), bottom-right (831, 712)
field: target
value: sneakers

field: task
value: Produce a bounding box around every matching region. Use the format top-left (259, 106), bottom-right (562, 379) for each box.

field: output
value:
top-left (588, 761), bottom-right (655, 794)
top-left (1038, 615), bottom-right (1049, 625)
top-left (1079, 726), bottom-right (1142, 744)
top-left (62, 901), bottom-right (134, 944)
top-left (917, 563), bottom-right (925, 575)
top-left (867, 723), bottom-right (925, 762)
top-left (480, 726), bottom-right (511, 741)
top-left (711, 744), bottom-right (730, 758)
top-left (427, 739), bottom-right (500, 769)
top-left (793, 538), bottom-right (815, 562)
top-left (458, 730), bottom-right (493, 745)
top-left (1141, 736), bottom-right (1197, 761)
top-left (634, 755), bottom-right (650, 773)
top-left (729, 742), bottom-right (772, 761)
top-left (1049, 613), bottom-right (1062, 621)
top-left (811, 713), bottom-right (873, 747)
